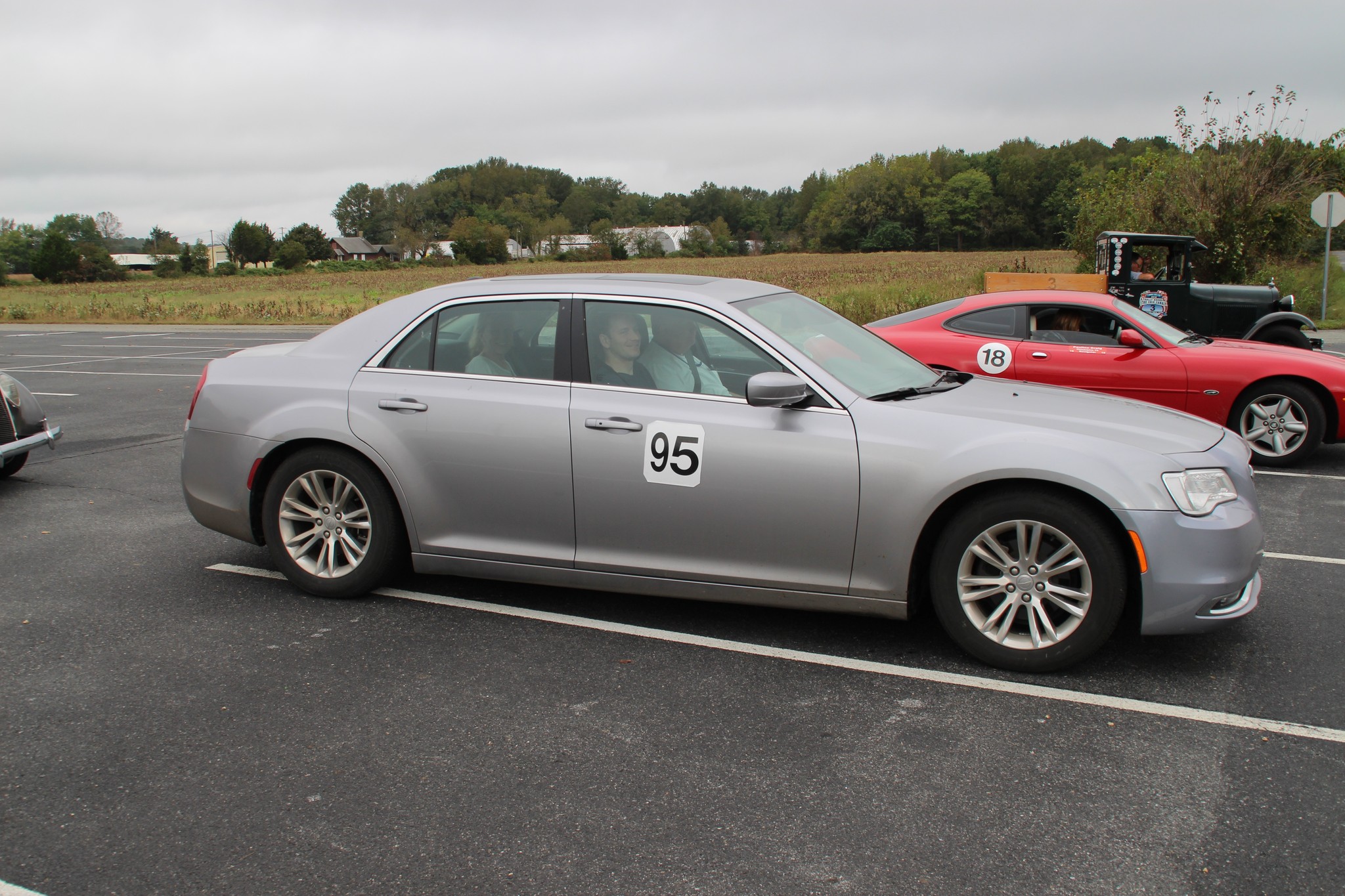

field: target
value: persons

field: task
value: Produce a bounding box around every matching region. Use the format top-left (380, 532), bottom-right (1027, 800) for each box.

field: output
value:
top-left (588, 307), bottom-right (735, 397)
top-left (1130, 252), bottom-right (1155, 281)
top-left (466, 309), bottom-right (520, 379)
top-left (1043, 308), bottom-right (1087, 345)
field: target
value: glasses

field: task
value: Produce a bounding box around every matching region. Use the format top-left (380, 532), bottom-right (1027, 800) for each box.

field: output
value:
top-left (1132, 259), bottom-right (1141, 267)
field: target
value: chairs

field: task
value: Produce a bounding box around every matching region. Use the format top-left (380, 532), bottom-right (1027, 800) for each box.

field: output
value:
top-left (590, 314), bottom-right (649, 377)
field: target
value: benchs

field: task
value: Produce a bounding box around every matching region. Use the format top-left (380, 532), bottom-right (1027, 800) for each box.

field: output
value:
top-left (390, 320), bottom-right (548, 379)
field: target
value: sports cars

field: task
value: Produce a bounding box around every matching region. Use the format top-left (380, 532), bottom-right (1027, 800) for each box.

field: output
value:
top-left (804, 288), bottom-right (1345, 470)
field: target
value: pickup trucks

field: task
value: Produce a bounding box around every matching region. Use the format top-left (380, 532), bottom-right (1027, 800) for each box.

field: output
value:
top-left (983, 229), bottom-right (1324, 352)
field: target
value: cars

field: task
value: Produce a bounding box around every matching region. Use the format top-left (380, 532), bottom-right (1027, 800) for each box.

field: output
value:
top-left (180, 275), bottom-right (1268, 673)
top-left (0, 371), bottom-right (66, 482)
